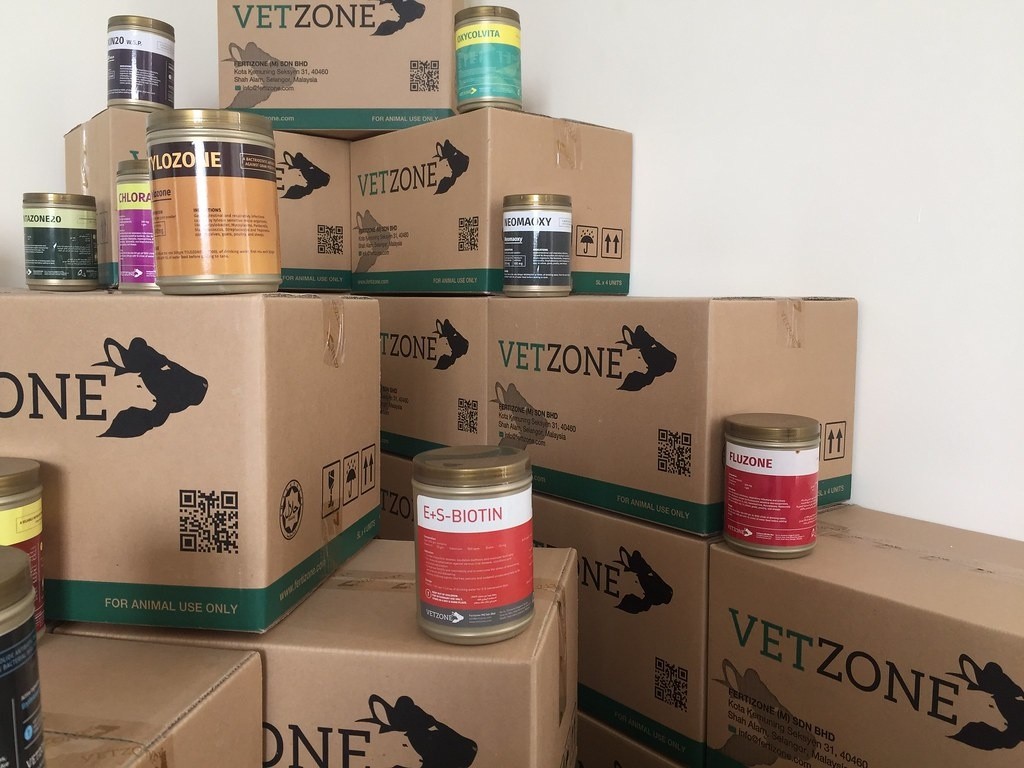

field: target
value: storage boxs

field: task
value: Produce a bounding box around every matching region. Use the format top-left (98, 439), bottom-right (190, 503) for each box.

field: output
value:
top-left (216, 0), bottom-right (455, 137)
top-left (488, 297), bottom-right (858, 537)
top-left (576, 713), bottom-right (684, 768)
top-left (0, 291), bottom-right (381, 635)
top-left (35, 634), bottom-right (262, 768)
top-left (42, 540), bottom-right (579, 768)
top-left (380, 296), bottom-right (488, 460)
top-left (532, 492), bottom-right (851, 768)
top-left (379, 452), bottom-right (415, 540)
top-left (350, 108), bottom-right (633, 297)
top-left (706, 504), bottom-right (1024, 768)
top-left (63, 107), bottom-right (350, 291)
